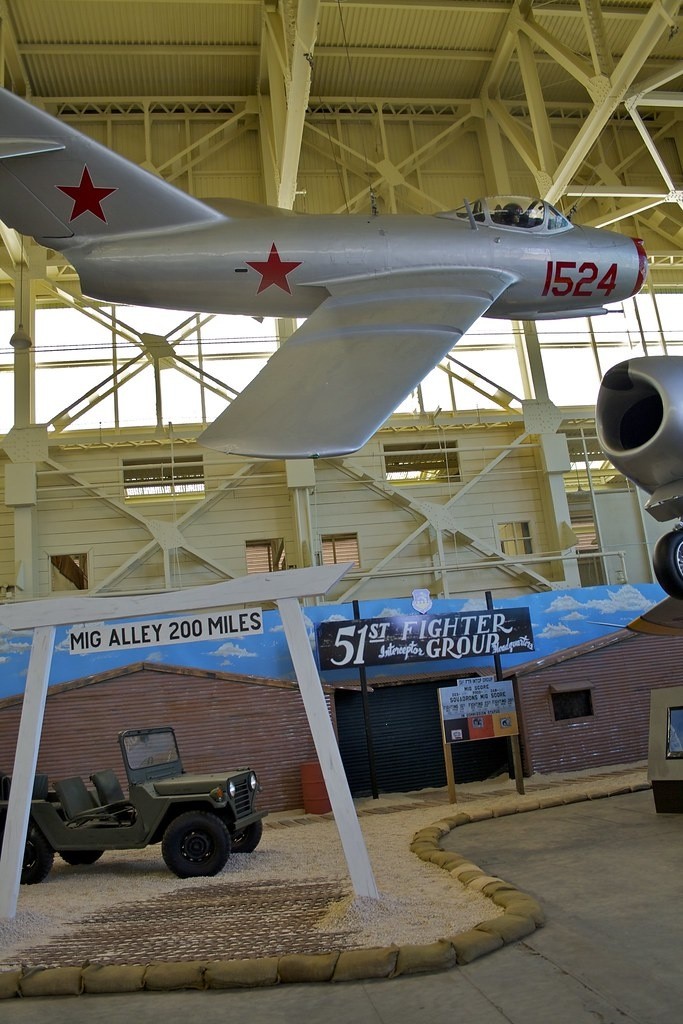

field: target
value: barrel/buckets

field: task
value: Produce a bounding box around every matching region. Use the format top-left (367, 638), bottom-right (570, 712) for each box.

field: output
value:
top-left (301, 761), bottom-right (331, 814)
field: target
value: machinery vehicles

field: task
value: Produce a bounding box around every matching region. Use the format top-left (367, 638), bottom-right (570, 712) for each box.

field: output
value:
top-left (0, 726), bottom-right (273, 880)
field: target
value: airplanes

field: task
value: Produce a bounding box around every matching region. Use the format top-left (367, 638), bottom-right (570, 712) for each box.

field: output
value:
top-left (595, 354), bottom-right (683, 599)
top-left (2, 79), bottom-right (650, 459)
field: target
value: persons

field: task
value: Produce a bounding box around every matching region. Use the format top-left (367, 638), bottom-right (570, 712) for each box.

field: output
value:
top-left (503, 203), bottom-right (524, 225)
